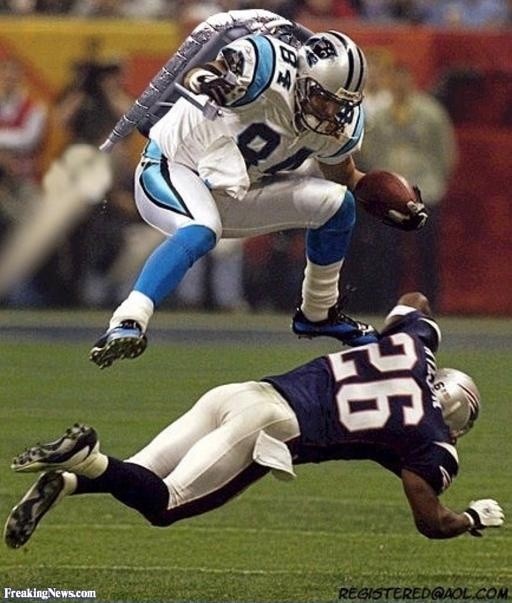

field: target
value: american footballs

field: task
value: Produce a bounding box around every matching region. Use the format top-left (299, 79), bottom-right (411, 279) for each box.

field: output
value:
top-left (355, 169), bottom-right (417, 223)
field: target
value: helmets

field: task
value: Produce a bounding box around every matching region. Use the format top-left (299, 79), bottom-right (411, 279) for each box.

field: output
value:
top-left (292, 29), bottom-right (368, 136)
top-left (433, 368), bottom-right (481, 438)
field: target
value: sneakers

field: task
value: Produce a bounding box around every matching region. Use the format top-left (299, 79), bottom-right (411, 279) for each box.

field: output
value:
top-left (9, 423), bottom-right (98, 473)
top-left (289, 301), bottom-right (379, 346)
top-left (5, 471), bottom-right (66, 550)
top-left (89, 318), bottom-right (147, 370)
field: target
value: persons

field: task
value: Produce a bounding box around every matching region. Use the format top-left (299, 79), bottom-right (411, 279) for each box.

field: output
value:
top-left (3, 291), bottom-right (506, 554)
top-left (0, 0), bottom-right (511, 307)
top-left (84, 27), bottom-right (431, 368)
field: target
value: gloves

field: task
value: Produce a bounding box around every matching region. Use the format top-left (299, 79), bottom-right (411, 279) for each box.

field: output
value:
top-left (380, 185), bottom-right (429, 231)
top-left (188, 68), bottom-right (236, 106)
top-left (464, 498), bottom-right (505, 530)
